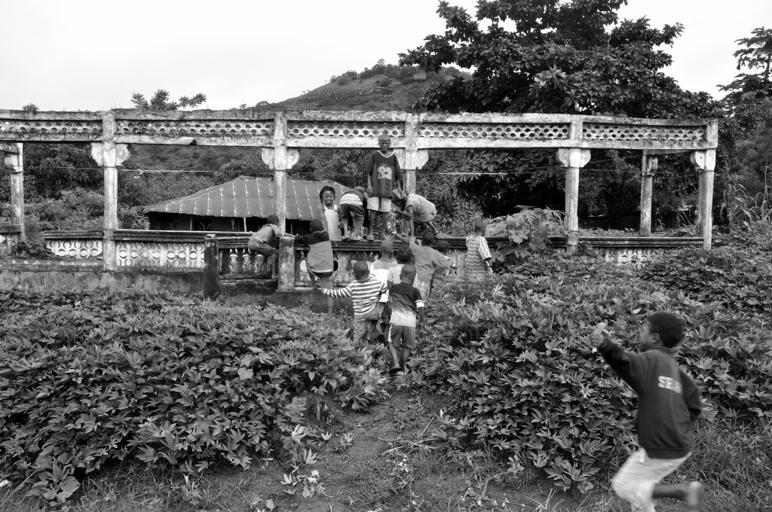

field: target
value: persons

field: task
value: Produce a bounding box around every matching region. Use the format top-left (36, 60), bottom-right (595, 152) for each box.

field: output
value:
top-left (247, 215), bottom-right (292, 275)
top-left (292, 186), bottom-right (454, 312)
top-left (385, 264), bottom-right (426, 373)
top-left (318, 260), bottom-right (386, 344)
top-left (363, 134), bottom-right (403, 242)
top-left (461, 219), bottom-right (493, 280)
top-left (586, 311), bottom-right (706, 511)
